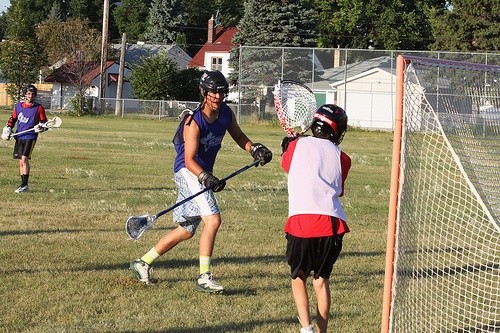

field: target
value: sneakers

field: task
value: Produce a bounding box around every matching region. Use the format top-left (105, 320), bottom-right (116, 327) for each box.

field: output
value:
top-left (15, 186), bottom-right (29, 193)
top-left (197, 272), bottom-right (224, 294)
top-left (129, 258), bottom-right (154, 286)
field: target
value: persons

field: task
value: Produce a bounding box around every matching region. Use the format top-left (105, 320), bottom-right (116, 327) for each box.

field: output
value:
top-left (280, 104), bottom-right (351, 333)
top-left (130, 70), bottom-right (272, 294)
top-left (1, 85), bottom-right (50, 193)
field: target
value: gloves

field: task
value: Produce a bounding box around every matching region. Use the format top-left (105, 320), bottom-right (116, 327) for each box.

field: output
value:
top-left (1, 127), bottom-right (13, 141)
top-left (250, 142), bottom-right (272, 167)
top-left (34, 124), bottom-right (46, 133)
top-left (198, 171), bottom-right (225, 193)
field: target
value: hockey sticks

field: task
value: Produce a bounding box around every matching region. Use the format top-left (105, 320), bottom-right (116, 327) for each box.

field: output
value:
top-left (274, 79), bottom-right (317, 137)
top-left (125, 160), bottom-right (262, 241)
top-left (9, 116), bottom-right (62, 137)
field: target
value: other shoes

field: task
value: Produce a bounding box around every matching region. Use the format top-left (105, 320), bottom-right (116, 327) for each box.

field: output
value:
top-left (301, 327), bottom-right (314, 333)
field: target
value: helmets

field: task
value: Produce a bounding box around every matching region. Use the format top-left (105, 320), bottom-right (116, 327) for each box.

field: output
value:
top-left (311, 104), bottom-right (347, 147)
top-left (25, 85), bottom-right (37, 93)
top-left (199, 70), bottom-right (229, 97)
